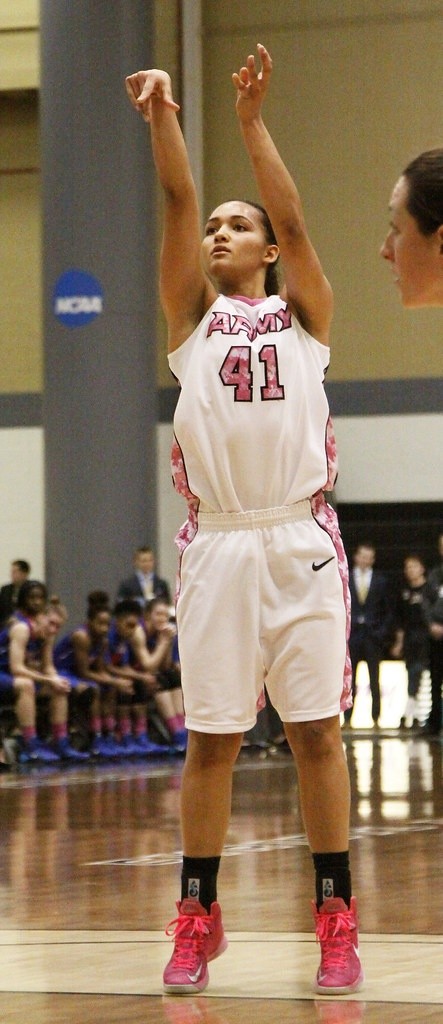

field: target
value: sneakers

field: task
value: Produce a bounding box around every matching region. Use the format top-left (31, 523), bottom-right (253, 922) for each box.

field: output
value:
top-left (161, 899), bottom-right (229, 995)
top-left (312, 896), bottom-right (366, 997)
top-left (19, 731), bottom-right (187, 765)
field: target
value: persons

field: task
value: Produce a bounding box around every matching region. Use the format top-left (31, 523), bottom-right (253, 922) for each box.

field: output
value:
top-left (125, 42), bottom-right (368, 992)
top-left (375, 147), bottom-right (443, 312)
top-left (0, 545), bottom-right (188, 768)
top-left (346, 526), bottom-right (443, 737)
